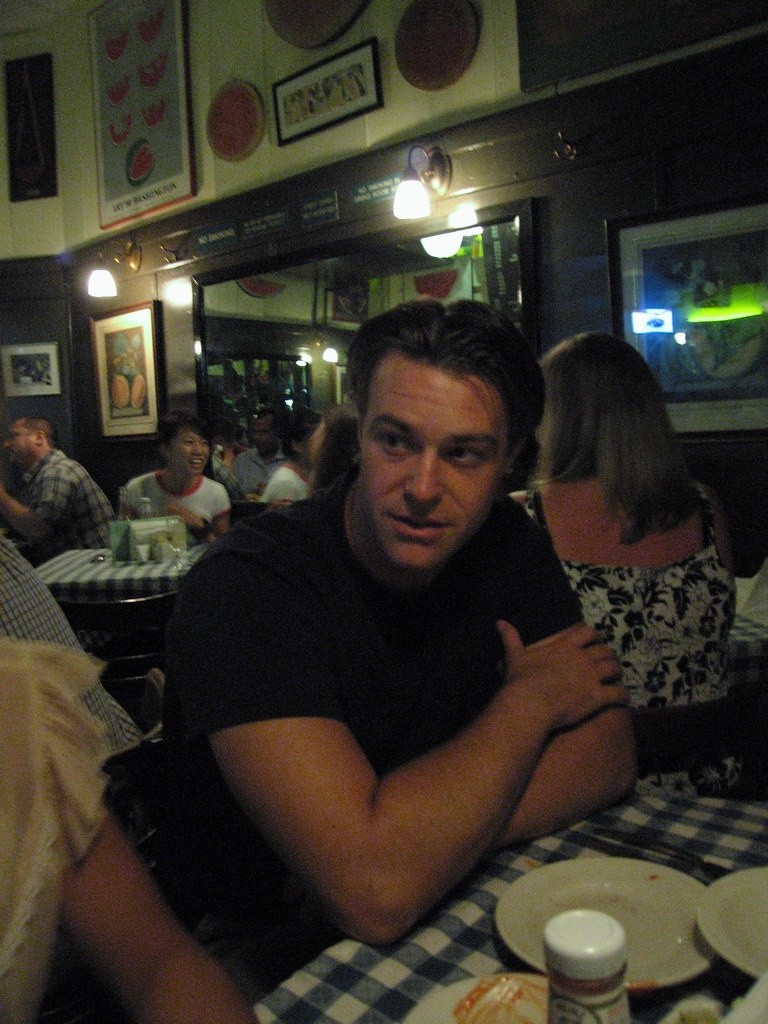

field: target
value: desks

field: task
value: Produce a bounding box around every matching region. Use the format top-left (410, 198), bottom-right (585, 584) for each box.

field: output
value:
top-left (36, 547), bottom-right (205, 647)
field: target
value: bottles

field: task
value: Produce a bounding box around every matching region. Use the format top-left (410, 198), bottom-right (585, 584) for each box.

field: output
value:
top-left (118, 486), bottom-right (153, 520)
top-left (544, 908), bottom-right (635, 1024)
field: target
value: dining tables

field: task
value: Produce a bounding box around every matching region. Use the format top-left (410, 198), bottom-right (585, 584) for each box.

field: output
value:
top-left (241, 781), bottom-right (768, 1024)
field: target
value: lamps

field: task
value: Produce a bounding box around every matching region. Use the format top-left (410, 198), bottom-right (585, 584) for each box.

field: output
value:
top-left (420, 225), bottom-right (480, 258)
top-left (313, 331), bottom-right (338, 363)
top-left (394, 144), bottom-right (452, 218)
top-left (87, 237), bottom-right (141, 297)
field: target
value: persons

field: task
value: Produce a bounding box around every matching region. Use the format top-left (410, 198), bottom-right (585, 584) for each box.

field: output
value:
top-left (0, 416), bottom-right (118, 560)
top-left (305, 409), bottom-right (360, 492)
top-left (0, 531), bottom-right (144, 758)
top-left (125, 300), bottom-right (638, 1002)
top-left (210, 409), bottom-right (323, 502)
top-left (510, 332), bottom-right (742, 796)
top-left (0, 637), bottom-right (259, 1024)
top-left (116, 410), bottom-right (232, 543)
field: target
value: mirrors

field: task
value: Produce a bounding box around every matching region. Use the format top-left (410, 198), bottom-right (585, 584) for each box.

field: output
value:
top-left (191, 192), bottom-right (540, 481)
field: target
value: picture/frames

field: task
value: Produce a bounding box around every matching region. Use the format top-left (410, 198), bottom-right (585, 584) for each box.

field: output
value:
top-left (84, 300), bottom-right (169, 446)
top-left (324, 289), bottom-right (367, 321)
top-left (272, 37), bottom-right (384, 147)
top-left (604, 196), bottom-right (768, 404)
top-left (0, 342), bottom-right (63, 400)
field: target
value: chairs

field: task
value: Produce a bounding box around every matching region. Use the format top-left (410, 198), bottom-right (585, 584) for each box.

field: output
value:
top-left (55, 595), bottom-right (238, 933)
top-left (628, 681), bottom-right (768, 804)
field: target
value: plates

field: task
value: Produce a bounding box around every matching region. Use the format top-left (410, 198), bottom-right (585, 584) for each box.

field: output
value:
top-left (698, 867), bottom-right (768, 978)
top-left (402, 972), bottom-right (549, 1024)
top-left (495, 858), bottom-right (714, 988)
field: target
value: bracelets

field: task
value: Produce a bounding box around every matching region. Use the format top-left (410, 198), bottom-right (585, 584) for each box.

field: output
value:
top-left (191, 519), bottom-right (212, 540)
